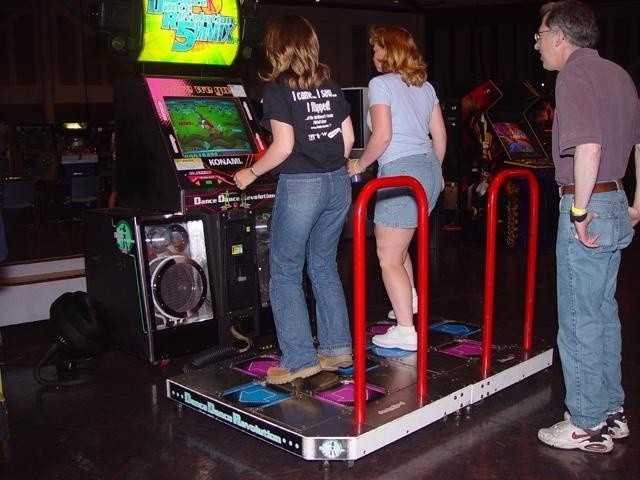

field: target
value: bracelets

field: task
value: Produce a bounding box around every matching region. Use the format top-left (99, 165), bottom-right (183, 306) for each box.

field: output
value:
top-left (250, 166), bottom-right (260, 179)
top-left (571, 203), bottom-right (588, 215)
top-left (570, 210), bottom-right (587, 223)
top-left (353, 159), bottom-right (365, 176)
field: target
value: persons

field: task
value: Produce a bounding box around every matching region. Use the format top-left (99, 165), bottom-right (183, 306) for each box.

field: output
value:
top-left (232, 10), bottom-right (356, 388)
top-left (219, 190), bottom-right (230, 206)
top-left (106, 130), bottom-right (118, 209)
top-left (531, 1), bottom-right (639, 457)
top-left (346, 16), bottom-right (449, 353)
top-left (119, 225), bottom-right (129, 251)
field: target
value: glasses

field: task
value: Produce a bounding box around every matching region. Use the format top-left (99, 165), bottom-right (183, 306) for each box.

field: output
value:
top-left (534, 29), bottom-right (565, 42)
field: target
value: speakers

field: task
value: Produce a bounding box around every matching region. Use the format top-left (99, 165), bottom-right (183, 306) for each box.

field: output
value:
top-left (81, 205), bottom-right (227, 366)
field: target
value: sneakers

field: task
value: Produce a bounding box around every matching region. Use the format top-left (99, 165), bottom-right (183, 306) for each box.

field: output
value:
top-left (371, 325), bottom-right (417, 351)
top-left (316, 353), bottom-right (354, 372)
top-left (266, 360), bottom-right (323, 385)
top-left (387, 296), bottom-right (418, 320)
top-left (537, 418), bottom-right (615, 451)
top-left (564, 407), bottom-right (630, 439)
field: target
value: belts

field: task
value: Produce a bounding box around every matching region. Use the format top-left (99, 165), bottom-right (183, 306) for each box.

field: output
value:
top-left (559, 181), bottom-right (625, 198)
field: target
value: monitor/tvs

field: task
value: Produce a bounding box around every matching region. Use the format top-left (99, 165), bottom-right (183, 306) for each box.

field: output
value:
top-left (491, 117), bottom-right (540, 159)
top-left (160, 95), bottom-right (259, 158)
